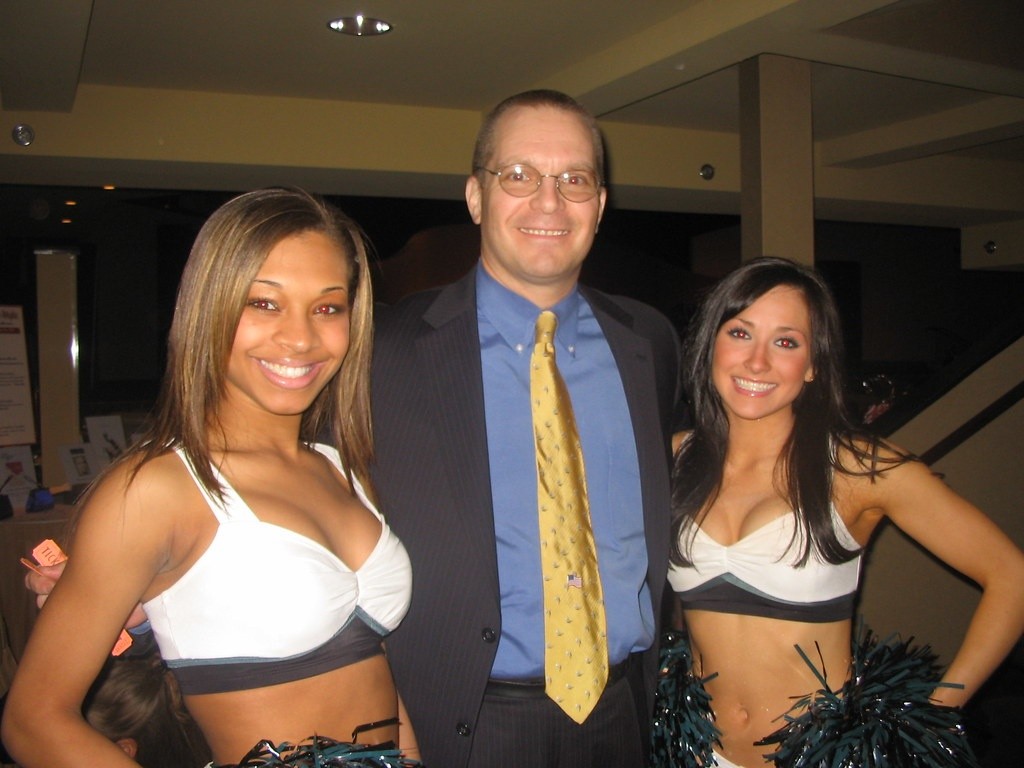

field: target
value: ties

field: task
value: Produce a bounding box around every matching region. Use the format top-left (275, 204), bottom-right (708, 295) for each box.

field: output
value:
top-left (529, 311), bottom-right (610, 725)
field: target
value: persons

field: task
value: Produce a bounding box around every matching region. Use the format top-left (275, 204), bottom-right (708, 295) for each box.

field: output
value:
top-left (0, 188), bottom-right (422, 768)
top-left (672, 256), bottom-right (1024, 768)
top-left (24, 89), bottom-right (699, 768)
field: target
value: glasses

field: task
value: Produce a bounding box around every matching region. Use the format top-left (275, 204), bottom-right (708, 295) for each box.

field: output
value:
top-left (471, 162), bottom-right (602, 203)
